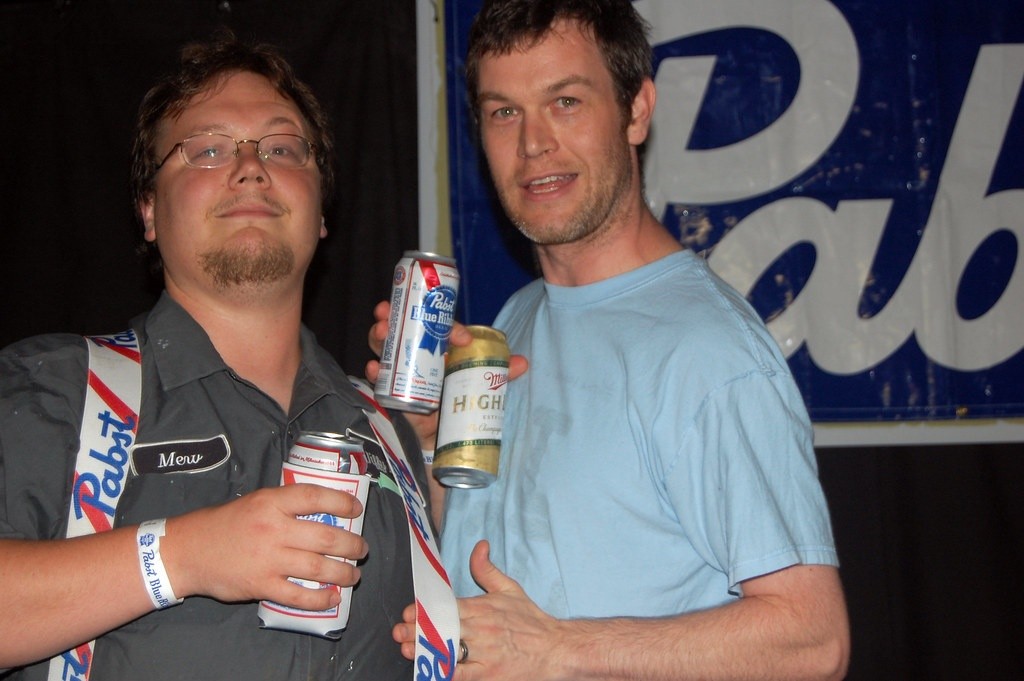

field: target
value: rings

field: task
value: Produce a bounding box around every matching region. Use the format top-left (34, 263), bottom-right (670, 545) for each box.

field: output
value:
top-left (457, 639), bottom-right (469, 663)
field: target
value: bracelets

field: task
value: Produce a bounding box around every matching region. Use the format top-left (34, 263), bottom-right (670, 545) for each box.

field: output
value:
top-left (421, 449), bottom-right (434, 464)
top-left (137, 517), bottom-right (184, 610)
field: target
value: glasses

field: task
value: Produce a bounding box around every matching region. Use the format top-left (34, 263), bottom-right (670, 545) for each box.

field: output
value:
top-left (146, 131), bottom-right (321, 188)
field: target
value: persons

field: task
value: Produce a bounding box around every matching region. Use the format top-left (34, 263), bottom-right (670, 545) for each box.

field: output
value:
top-left (0, 40), bottom-right (440, 681)
top-left (366, 0), bottom-right (851, 681)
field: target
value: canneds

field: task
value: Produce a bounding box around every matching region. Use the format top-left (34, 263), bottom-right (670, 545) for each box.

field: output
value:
top-left (432, 324), bottom-right (510, 489)
top-left (258, 430), bottom-right (370, 639)
top-left (374, 250), bottom-right (460, 414)
